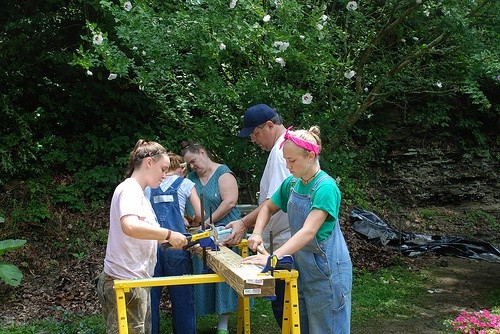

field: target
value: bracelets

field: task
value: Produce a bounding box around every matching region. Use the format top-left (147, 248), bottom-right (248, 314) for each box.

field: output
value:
top-left (240, 218), bottom-right (250, 229)
top-left (165, 229), bottom-right (172, 240)
top-left (253, 230), bottom-right (263, 236)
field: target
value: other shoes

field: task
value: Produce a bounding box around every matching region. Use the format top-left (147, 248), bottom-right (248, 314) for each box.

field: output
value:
top-left (217, 329), bottom-right (229, 334)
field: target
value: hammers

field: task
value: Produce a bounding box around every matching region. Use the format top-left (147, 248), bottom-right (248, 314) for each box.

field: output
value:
top-left (184, 213), bottom-right (202, 228)
top-left (246, 233), bottom-right (270, 257)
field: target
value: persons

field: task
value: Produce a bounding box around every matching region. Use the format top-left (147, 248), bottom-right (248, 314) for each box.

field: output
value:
top-left (240, 125), bottom-right (352, 334)
top-left (96, 139), bottom-right (223, 334)
top-left (224, 104), bottom-right (321, 330)
top-left (178, 140), bottom-right (254, 334)
top-left (143, 151), bottom-right (206, 334)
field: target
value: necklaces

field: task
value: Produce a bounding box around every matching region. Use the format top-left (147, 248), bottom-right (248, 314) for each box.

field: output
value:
top-left (300, 169), bottom-right (320, 186)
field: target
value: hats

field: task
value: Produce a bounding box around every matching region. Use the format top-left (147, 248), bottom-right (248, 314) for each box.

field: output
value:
top-left (239, 104), bottom-right (276, 137)
